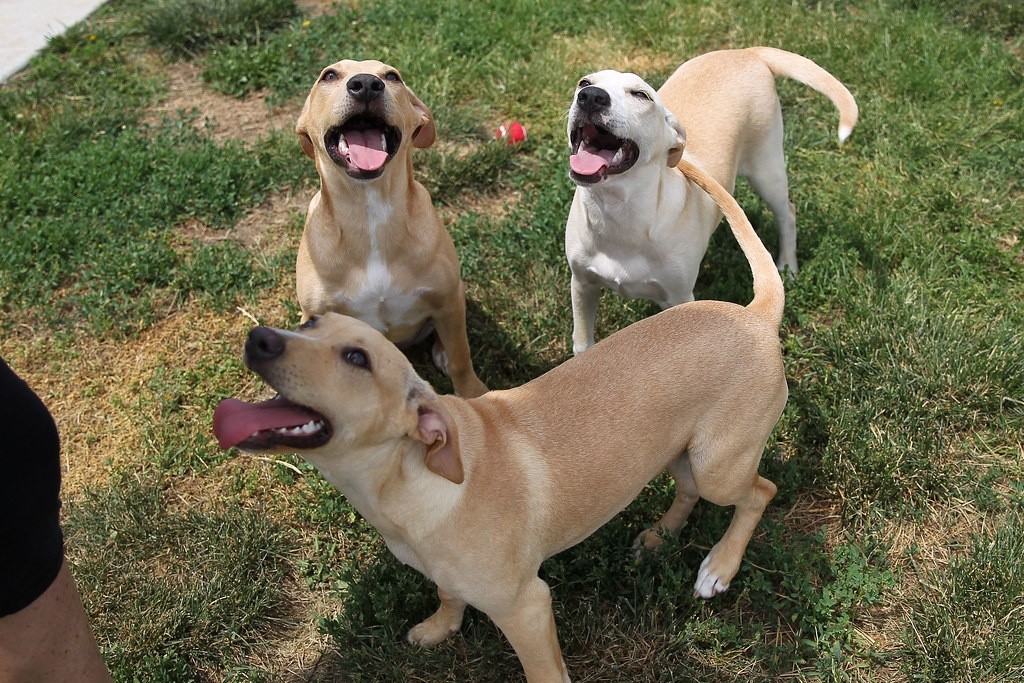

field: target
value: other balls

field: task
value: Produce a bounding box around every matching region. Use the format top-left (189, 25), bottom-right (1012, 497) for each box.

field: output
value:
top-left (496, 121), bottom-right (529, 146)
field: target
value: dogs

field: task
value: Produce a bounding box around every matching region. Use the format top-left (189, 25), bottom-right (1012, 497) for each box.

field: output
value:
top-left (293, 58), bottom-right (490, 401)
top-left (563, 45), bottom-right (859, 358)
top-left (210, 159), bottom-right (790, 683)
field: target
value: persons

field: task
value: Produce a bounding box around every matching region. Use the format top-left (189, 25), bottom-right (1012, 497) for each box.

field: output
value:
top-left (0, 357), bottom-right (117, 683)
